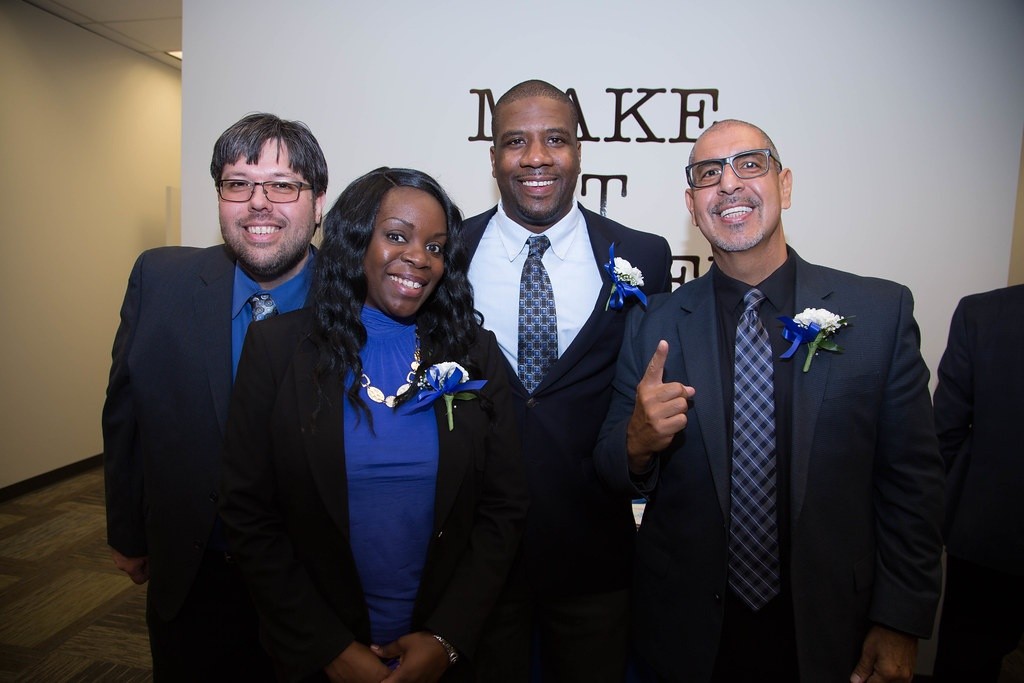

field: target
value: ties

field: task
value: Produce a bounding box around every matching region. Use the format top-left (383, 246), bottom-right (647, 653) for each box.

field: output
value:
top-left (727, 289), bottom-right (781, 615)
top-left (249, 292), bottom-right (276, 321)
top-left (518, 235), bottom-right (558, 394)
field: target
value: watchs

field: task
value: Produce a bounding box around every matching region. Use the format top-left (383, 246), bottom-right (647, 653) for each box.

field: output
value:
top-left (433, 633), bottom-right (458, 666)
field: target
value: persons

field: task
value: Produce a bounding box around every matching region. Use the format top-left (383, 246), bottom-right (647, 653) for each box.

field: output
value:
top-left (599, 121), bottom-right (945, 683)
top-left (298, 167), bottom-right (529, 683)
top-left (458, 79), bottom-right (674, 683)
top-left (102, 119), bottom-right (329, 683)
top-left (934, 282), bottom-right (1024, 682)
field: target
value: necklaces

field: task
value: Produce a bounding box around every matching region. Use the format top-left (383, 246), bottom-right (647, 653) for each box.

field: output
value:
top-left (357, 327), bottom-right (421, 409)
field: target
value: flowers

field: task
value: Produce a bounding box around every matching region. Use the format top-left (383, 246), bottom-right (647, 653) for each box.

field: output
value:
top-left (602, 243), bottom-right (648, 314)
top-left (778, 306), bottom-right (856, 371)
top-left (399, 359), bottom-right (489, 432)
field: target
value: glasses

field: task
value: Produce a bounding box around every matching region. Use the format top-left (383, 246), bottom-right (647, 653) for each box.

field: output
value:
top-left (685, 149), bottom-right (782, 188)
top-left (216, 179), bottom-right (313, 204)
